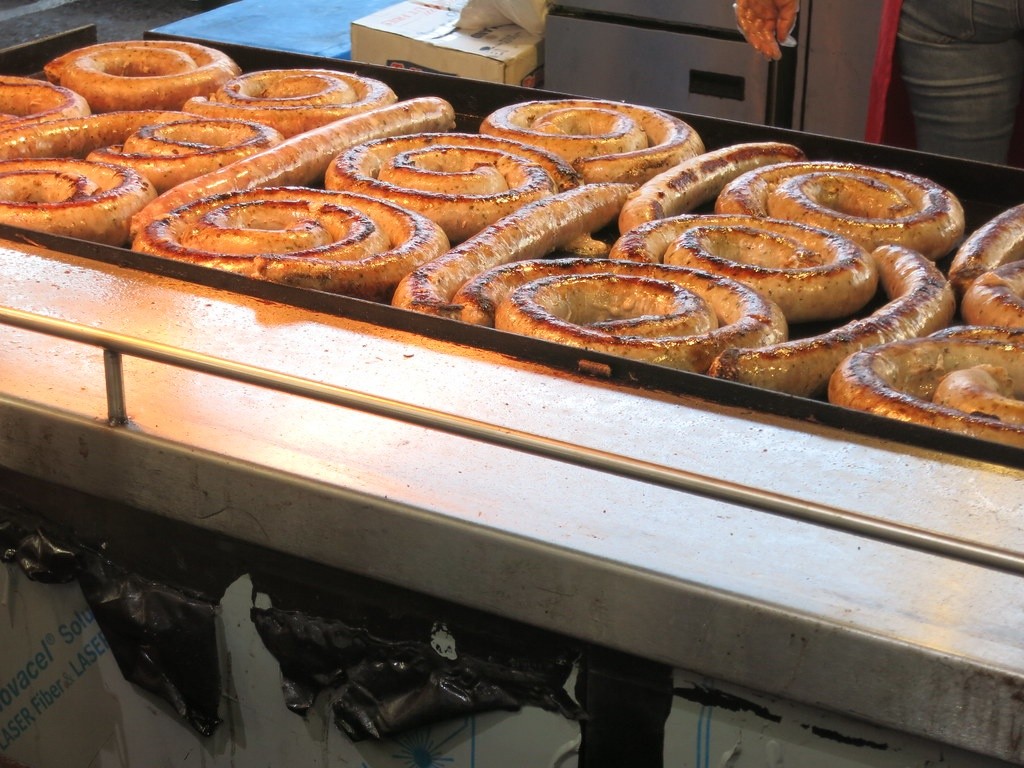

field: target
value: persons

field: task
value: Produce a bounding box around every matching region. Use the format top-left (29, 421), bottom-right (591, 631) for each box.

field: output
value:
top-left (732, 0), bottom-right (1024, 170)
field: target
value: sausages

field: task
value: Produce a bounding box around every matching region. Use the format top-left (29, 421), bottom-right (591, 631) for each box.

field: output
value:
top-left (0, 39), bottom-right (1024, 450)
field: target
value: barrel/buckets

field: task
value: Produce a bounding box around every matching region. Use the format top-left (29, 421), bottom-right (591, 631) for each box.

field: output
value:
top-left (900, 0), bottom-right (1022, 165)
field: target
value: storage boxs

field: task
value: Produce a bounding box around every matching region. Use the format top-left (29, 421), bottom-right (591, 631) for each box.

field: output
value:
top-left (351, 1), bottom-right (543, 93)
